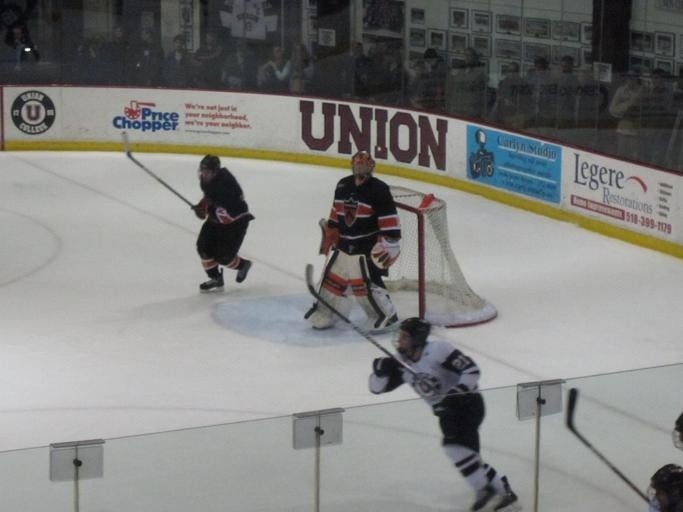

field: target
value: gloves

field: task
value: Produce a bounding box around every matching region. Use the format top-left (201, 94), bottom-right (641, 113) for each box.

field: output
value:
top-left (191, 201), bottom-right (208, 219)
top-left (373, 358), bottom-right (396, 379)
top-left (431, 397), bottom-right (458, 417)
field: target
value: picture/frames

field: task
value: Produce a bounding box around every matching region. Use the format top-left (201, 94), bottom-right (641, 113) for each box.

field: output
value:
top-left (169, 2), bottom-right (680, 97)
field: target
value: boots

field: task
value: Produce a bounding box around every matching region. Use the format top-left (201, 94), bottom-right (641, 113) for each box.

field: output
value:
top-left (493, 476), bottom-right (516, 511)
top-left (472, 486), bottom-right (494, 511)
top-left (199, 268), bottom-right (223, 289)
top-left (234, 258), bottom-right (251, 282)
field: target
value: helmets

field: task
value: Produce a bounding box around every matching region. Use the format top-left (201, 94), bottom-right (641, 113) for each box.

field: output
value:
top-left (392, 317), bottom-right (430, 359)
top-left (199, 154), bottom-right (220, 171)
top-left (672, 413), bottom-right (682, 451)
top-left (351, 150), bottom-right (374, 180)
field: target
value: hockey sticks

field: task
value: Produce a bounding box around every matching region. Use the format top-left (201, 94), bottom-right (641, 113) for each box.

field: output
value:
top-left (306, 263), bottom-right (445, 399)
top-left (121, 132), bottom-right (197, 206)
top-left (568, 387), bottom-right (651, 504)
top-left (305, 246), bottom-right (335, 318)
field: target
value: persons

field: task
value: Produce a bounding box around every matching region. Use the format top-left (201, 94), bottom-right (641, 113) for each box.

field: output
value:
top-left (366, 316), bottom-right (524, 512)
top-left (8, 22), bottom-right (258, 91)
top-left (299, 148), bottom-right (403, 335)
top-left (258, 36), bottom-right (486, 122)
top-left (671, 412), bottom-right (683, 451)
top-left (190, 154), bottom-right (255, 296)
top-left (490, 55), bottom-right (681, 173)
